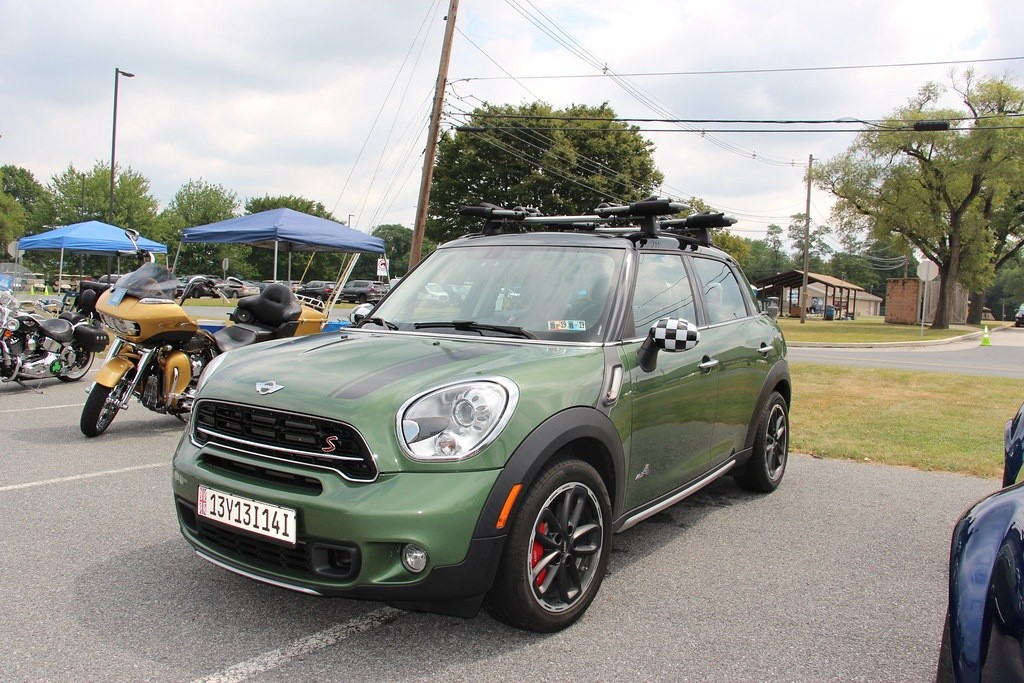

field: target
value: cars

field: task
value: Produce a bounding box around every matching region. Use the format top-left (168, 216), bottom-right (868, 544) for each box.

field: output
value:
top-left (1012, 305), bottom-right (1023, 326)
top-left (173, 196), bottom-right (791, 633)
top-left (100, 267), bottom-right (385, 307)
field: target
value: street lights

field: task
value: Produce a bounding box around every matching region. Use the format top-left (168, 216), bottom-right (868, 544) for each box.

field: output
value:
top-left (110, 67), bottom-right (132, 230)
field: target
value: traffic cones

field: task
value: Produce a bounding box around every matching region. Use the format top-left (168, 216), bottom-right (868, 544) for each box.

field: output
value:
top-left (982, 325), bottom-right (992, 347)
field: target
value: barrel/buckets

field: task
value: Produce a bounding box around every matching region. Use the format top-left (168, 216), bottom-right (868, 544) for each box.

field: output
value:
top-left (826, 309), bottom-right (834, 320)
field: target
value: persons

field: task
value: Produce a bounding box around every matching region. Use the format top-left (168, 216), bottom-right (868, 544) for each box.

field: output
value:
top-left (812, 297), bottom-right (817, 312)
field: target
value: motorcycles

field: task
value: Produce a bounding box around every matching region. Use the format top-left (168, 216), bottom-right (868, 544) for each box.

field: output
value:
top-left (2, 285), bottom-right (107, 382)
top-left (81, 230), bottom-right (339, 435)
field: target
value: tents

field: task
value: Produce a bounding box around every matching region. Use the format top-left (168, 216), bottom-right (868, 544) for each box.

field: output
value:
top-left (14, 220), bottom-right (168, 293)
top-left (172, 208), bottom-right (391, 290)
